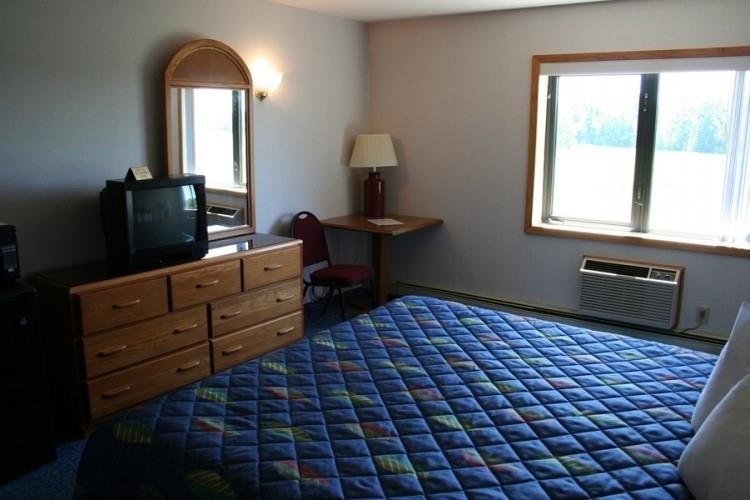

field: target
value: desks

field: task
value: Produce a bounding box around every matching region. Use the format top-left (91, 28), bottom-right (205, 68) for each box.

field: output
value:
top-left (29, 232), bottom-right (304, 440)
top-left (320, 214), bottom-right (444, 309)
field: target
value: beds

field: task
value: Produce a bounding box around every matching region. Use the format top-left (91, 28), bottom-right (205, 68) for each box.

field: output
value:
top-left (72, 293), bottom-right (718, 500)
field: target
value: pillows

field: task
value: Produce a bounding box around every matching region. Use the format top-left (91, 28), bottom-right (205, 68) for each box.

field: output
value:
top-left (678, 301), bottom-right (750, 500)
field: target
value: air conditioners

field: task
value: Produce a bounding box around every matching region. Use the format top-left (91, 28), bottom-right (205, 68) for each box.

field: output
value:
top-left (577, 258), bottom-right (681, 329)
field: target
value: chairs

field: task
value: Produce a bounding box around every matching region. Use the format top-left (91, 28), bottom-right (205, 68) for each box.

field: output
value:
top-left (289, 211), bottom-right (375, 321)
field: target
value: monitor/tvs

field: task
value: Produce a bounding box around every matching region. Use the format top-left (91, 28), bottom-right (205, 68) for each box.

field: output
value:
top-left (99, 176), bottom-right (208, 271)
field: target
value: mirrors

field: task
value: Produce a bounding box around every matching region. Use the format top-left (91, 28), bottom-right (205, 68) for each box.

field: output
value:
top-left (165, 39), bottom-right (255, 241)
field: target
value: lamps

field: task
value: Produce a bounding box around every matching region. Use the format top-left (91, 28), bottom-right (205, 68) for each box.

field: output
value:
top-left (349, 134), bottom-right (399, 219)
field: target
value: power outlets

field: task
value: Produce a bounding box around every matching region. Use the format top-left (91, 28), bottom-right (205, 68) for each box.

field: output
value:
top-left (697, 305), bottom-right (710, 323)
